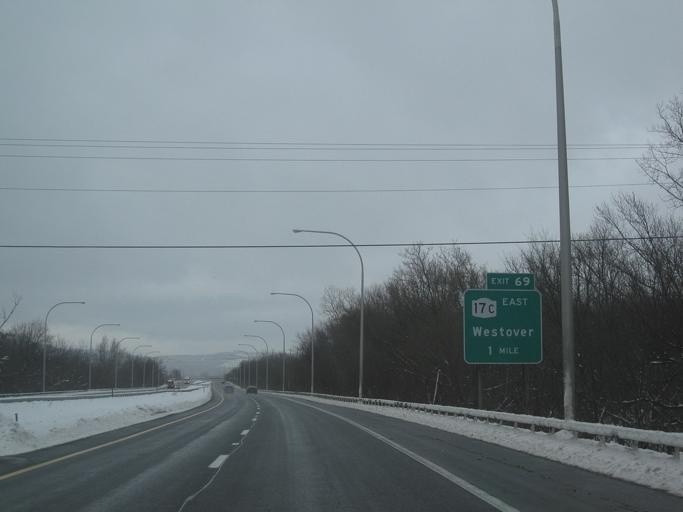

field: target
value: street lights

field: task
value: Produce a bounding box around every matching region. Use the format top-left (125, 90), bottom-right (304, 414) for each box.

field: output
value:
top-left (87, 323), bottom-right (120, 390)
top-left (292, 229), bottom-right (364, 399)
top-left (131, 344), bottom-right (151, 389)
top-left (41, 300), bottom-right (85, 394)
top-left (253, 320), bottom-right (285, 392)
top-left (270, 292), bottom-right (315, 395)
top-left (237, 343), bottom-right (258, 390)
top-left (142, 350), bottom-right (159, 388)
top-left (244, 334), bottom-right (269, 390)
top-left (114, 336), bottom-right (140, 389)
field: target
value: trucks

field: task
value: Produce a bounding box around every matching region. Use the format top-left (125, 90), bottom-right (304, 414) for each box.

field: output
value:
top-left (166, 377), bottom-right (179, 389)
top-left (183, 375), bottom-right (193, 384)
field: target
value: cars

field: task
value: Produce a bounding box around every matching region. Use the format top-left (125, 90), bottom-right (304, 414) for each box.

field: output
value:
top-left (246, 385), bottom-right (258, 394)
top-left (223, 384), bottom-right (233, 391)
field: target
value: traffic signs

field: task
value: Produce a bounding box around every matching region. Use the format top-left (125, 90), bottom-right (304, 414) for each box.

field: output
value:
top-left (485, 272), bottom-right (536, 289)
top-left (462, 289), bottom-right (544, 366)
